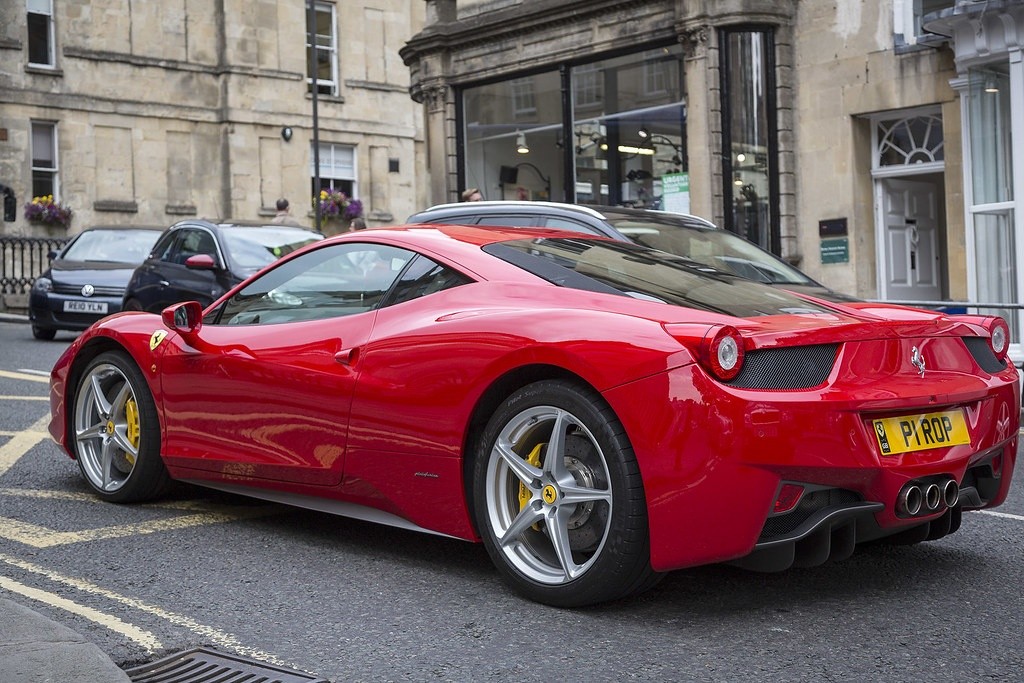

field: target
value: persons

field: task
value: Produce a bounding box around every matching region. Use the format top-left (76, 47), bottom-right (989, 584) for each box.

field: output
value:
top-left (270, 198), bottom-right (300, 226)
top-left (461, 188), bottom-right (481, 203)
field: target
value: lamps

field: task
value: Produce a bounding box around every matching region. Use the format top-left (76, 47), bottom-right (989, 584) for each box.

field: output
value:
top-left (515, 131), bottom-right (530, 154)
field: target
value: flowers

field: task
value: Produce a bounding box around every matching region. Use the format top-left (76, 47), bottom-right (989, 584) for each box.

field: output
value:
top-left (314, 186), bottom-right (362, 218)
top-left (24, 195), bottom-right (71, 229)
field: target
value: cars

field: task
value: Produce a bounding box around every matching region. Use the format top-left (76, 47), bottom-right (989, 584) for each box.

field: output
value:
top-left (26, 223), bottom-right (174, 343)
top-left (121, 221), bottom-right (364, 318)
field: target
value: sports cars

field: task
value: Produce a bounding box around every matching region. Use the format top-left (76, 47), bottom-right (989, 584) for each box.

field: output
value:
top-left (46, 222), bottom-right (1022, 610)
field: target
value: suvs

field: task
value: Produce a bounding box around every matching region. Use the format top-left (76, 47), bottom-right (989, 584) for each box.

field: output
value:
top-left (404, 199), bottom-right (832, 292)
